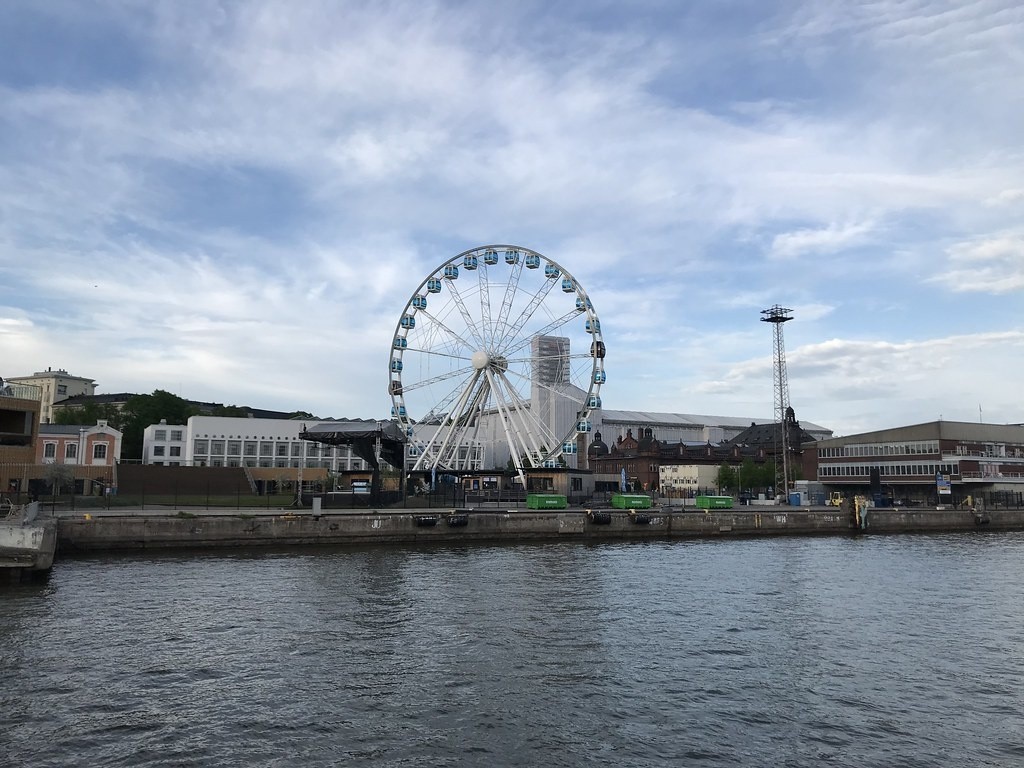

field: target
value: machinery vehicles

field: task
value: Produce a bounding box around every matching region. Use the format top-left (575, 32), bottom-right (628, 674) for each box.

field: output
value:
top-left (829, 491), bottom-right (844, 507)
